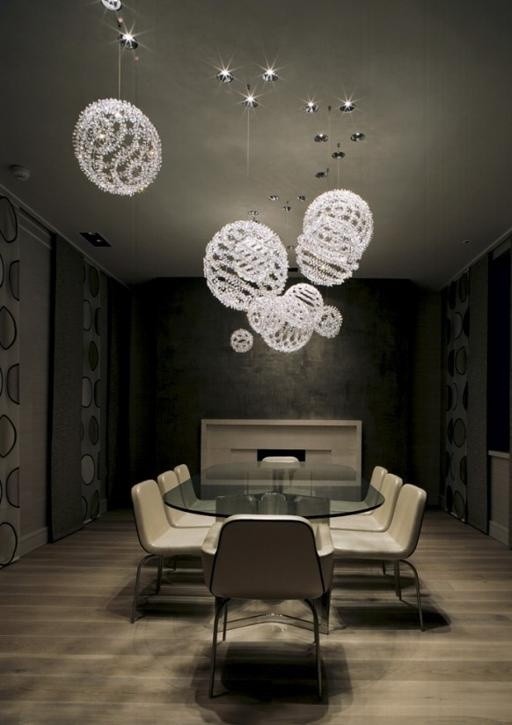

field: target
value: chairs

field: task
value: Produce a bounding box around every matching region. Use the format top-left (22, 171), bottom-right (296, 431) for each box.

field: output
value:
top-left (328, 465), bottom-right (428, 634)
top-left (262, 456), bottom-right (299, 462)
top-left (202, 513), bottom-right (334, 701)
top-left (128, 463), bottom-right (224, 623)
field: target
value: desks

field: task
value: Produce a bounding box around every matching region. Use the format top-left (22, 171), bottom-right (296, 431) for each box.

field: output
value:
top-left (166, 462), bottom-right (383, 636)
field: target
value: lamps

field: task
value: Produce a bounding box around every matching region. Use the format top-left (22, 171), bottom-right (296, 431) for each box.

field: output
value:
top-left (230, 282), bottom-right (342, 355)
top-left (202, 220), bottom-right (288, 312)
top-left (70, 35), bottom-right (163, 197)
top-left (294, 189), bottom-right (375, 282)
